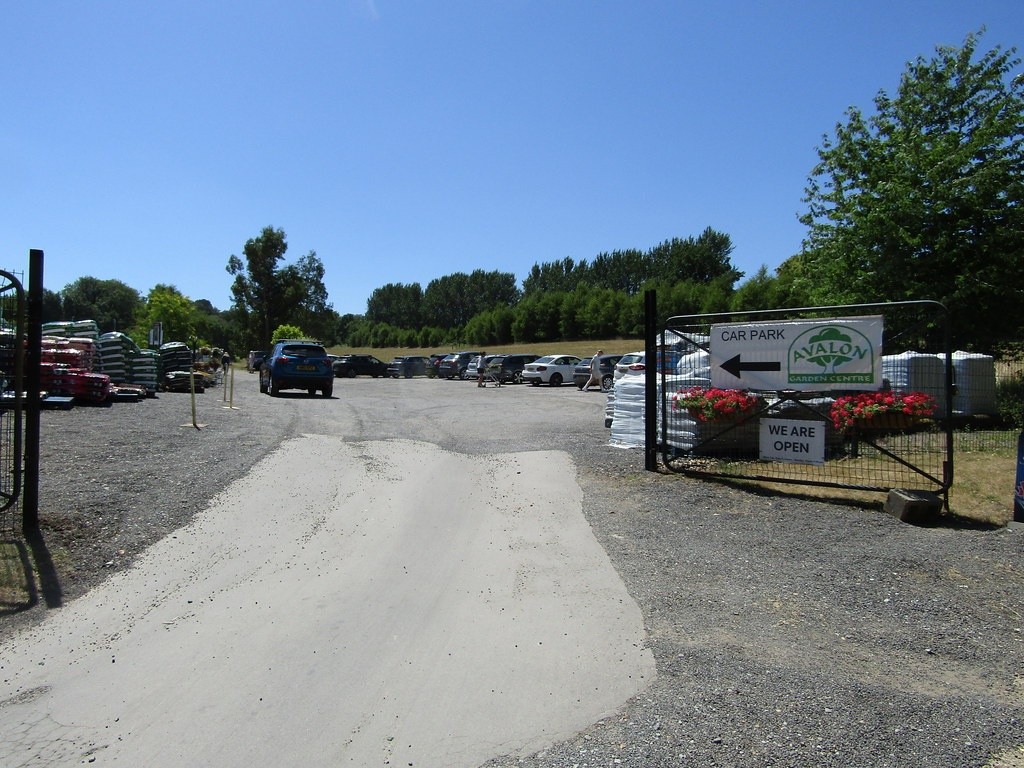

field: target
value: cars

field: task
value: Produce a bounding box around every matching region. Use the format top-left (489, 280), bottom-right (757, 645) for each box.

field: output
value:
top-left (467, 356), bottom-right (497, 380)
top-left (327, 353), bottom-right (338, 363)
top-left (523, 355), bottom-right (582, 386)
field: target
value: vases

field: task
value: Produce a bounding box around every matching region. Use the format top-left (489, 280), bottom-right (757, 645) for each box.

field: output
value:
top-left (857, 411), bottom-right (912, 430)
top-left (686, 406), bottom-right (736, 425)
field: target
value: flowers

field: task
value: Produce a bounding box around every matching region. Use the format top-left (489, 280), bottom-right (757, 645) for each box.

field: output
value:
top-left (828, 388), bottom-right (939, 438)
top-left (670, 386), bottom-right (760, 424)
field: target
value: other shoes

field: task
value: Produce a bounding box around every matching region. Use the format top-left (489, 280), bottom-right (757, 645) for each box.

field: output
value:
top-left (582, 388), bottom-right (588, 392)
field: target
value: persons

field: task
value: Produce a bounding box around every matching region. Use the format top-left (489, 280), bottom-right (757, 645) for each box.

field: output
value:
top-left (221, 352), bottom-right (230, 373)
top-left (582, 350), bottom-right (607, 392)
top-left (476, 352), bottom-right (486, 387)
top-left (248, 351), bottom-right (255, 374)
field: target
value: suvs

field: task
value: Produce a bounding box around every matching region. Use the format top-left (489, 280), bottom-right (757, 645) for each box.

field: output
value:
top-left (387, 355), bottom-right (431, 379)
top-left (440, 352), bottom-right (485, 380)
top-left (614, 352), bottom-right (648, 384)
top-left (261, 341), bottom-right (334, 398)
top-left (249, 351), bottom-right (265, 370)
top-left (489, 355), bottom-right (542, 383)
top-left (332, 355), bottom-right (390, 378)
top-left (572, 355), bottom-right (622, 391)
top-left (426, 354), bottom-right (449, 378)
